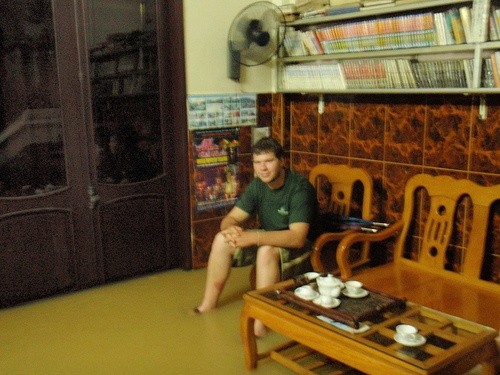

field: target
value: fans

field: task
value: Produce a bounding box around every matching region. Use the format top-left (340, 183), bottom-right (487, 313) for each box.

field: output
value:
top-left (227, 1), bottom-right (287, 80)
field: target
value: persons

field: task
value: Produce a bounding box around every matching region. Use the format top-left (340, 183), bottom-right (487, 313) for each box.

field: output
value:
top-left (106, 128), bottom-right (157, 186)
top-left (189, 136), bottom-right (318, 338)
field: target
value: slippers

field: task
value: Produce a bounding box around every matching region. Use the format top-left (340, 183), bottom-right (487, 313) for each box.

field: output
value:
top-left (190, 307), bottom-right (202, 317)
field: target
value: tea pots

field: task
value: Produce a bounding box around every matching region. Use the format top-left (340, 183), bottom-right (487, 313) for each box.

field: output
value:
top-left (316, 272), bottom-right (344, 297)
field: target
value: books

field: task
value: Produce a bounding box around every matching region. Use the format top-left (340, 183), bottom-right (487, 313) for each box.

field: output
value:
top-left (282, 28), bottom-right (323, 57)
top-left (88, 30), bottom-right (158, 114)
top-left (411, 51), bottom-right (500, 88)
top-left (313, 7), bottom-right (500, 53)
top-left (277, 0), bottom-right (441, 23)
top-left (281, 58), bottom-right (419, 90)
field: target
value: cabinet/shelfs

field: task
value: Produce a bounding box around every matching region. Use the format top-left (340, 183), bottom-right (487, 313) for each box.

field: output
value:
top-left (272, 0), bottom-right (500, 94)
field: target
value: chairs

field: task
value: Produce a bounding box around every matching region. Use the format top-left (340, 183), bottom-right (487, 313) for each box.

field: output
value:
top-left (249, 164), bottom-right (373, 275)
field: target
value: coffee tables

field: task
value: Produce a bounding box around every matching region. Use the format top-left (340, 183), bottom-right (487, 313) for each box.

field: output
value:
top-left (241, 276), bottom-right (497, 375)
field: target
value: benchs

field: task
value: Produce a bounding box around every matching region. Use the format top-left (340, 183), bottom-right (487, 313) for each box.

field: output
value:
top-left (336, 173), bottom-right (500, 341)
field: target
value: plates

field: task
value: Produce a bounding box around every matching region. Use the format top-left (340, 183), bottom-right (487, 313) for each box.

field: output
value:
top-left (342, 288), bottom-right (368, 299)
top-left (312, 297), bottom-right (342, 307)
top-left (394, 333), bottom-right (427, 347)
top-left (295, 286), bottom-right (319, 300)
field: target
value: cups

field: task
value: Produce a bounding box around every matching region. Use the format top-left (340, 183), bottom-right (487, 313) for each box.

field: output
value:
top-left (305, 272), bottom-right (320, 283)
top-left (346, 280), bottom-right (363, 294)
top-left (301, 285), bottom-right (315, 297)
top-left (396, 324), bottom-right (418, 342)
